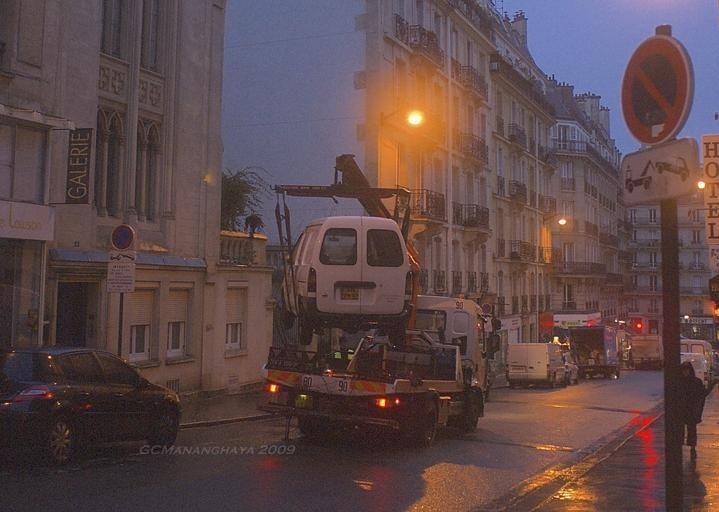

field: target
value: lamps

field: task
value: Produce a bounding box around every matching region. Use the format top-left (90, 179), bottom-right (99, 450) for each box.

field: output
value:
top-left (380, 95), bottom-right (421, 129)
top-left (544, 208), bottom-right (567, 225)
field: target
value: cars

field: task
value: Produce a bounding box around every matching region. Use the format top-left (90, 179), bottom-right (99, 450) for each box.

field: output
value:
top-left (0, 345), bottom-right (182, 471)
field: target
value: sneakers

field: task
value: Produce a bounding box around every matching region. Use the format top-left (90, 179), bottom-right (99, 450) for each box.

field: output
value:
top-left (690, 448), bottom-right (697, 459)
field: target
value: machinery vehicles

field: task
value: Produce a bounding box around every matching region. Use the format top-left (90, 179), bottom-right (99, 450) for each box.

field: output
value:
top-left (256, 148), bottom-right (501, 453)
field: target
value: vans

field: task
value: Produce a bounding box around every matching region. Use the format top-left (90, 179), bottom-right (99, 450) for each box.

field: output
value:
top-left (277, 217), bottom-right (415, 342)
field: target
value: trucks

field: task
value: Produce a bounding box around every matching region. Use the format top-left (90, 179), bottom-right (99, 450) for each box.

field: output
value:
top-left (505, 326), bottom-right (715, 393)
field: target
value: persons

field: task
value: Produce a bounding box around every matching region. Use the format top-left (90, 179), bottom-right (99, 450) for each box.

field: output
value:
top-left (681, 362), bottom-right (704, 458)
